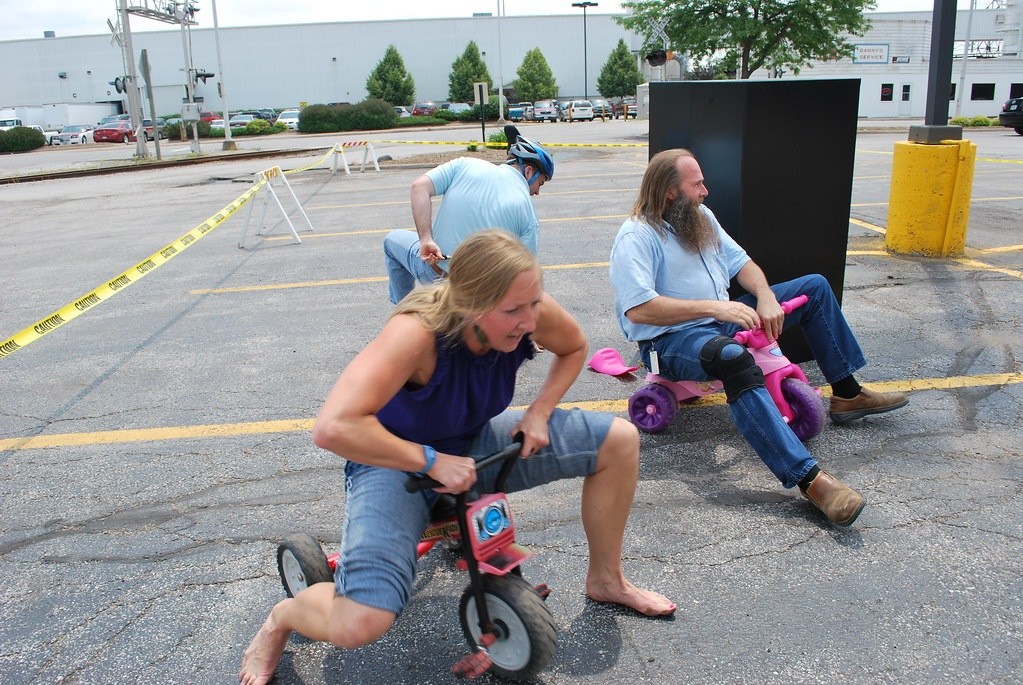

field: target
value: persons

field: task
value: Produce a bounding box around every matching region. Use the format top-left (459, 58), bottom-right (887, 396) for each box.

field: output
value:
top-left (383, 135), bottom-right (554, 306)
top-left (237, 228), bottom-right (677, 685)
top-left (609, 148), bottom-right (909, 527)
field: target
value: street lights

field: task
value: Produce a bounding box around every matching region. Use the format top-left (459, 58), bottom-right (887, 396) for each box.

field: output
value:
top-left (572, 1), bottom-right (598, 99)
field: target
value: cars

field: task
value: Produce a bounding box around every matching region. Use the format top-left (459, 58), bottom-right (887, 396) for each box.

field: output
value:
top-left (438, 102), bottom-right (450, 112)
top-left (999, 95), bottom-right (1023, 135)
top-left (0, 111), bottom-right (277, 141)
top-left (276, 109), bottom-right (301, 131)
top-left (393, 105), bottom-right (411, 118)
top-left (93, 120), bottom-right (148, 145)
top-left (591, 98), bottom-right (613, 120)
top-left (448, 102), bottom-right (471, 114)
top-left (615, 97), bottom-right (637, 119)
top-left (52, 123), bottom-right (96, 146)
top-left (607, 97), bottom-right (617, 118)
top-left (505, 99), bottom-right (594, 123)
top-left (413, 101), bottom-right (439, 117)
top-left (142, 117), bottom-right (170, 141)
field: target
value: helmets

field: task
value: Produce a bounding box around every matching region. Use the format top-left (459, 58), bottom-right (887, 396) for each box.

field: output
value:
top-left (507, 135), bottom-right (554, 181)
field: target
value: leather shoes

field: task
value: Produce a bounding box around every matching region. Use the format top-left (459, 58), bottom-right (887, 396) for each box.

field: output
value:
top-left (829, 386), bottom-right (909, 423)
top-left (799, 470), bottom-right (866, 526)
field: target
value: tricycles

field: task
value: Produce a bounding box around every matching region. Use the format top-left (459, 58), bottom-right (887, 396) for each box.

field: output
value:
top-left (627, 293), bottom-right (827, 444)
top-left (277, 430), bottom-right (560, 684)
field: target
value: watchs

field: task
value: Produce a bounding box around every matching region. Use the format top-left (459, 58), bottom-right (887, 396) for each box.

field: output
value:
top-left (414, 445), bottom-right (437, 478)
